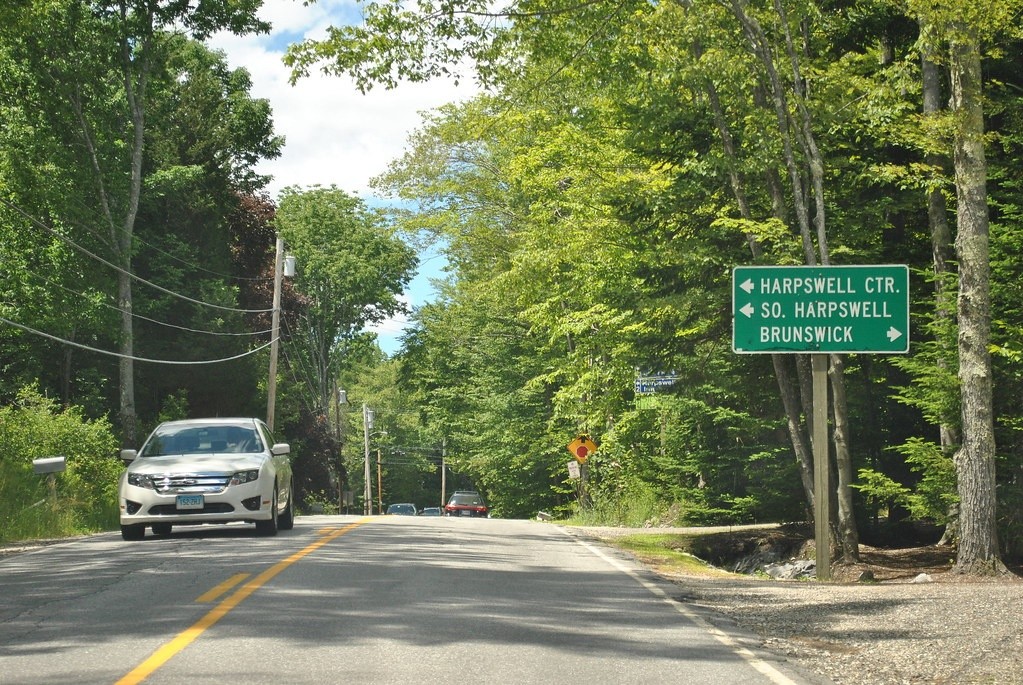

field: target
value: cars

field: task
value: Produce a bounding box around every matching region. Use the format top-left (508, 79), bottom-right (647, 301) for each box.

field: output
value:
top-left (118, 417), bottom-right (294, 541)
top-left (445, 491), bottom-right (487, 518)
top-left (386, 503), bottom-right (444, 516)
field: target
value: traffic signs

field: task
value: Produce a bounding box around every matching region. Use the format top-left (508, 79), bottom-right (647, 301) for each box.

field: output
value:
top-left (732, 264), bottom-right (910, 354)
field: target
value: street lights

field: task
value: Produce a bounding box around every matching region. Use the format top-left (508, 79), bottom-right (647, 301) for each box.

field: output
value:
top-left (362, 404), bottom-right (388, 515)
top-left (266, 232), bottom-right (295, 433)
top-left (332, 378), bottom-right (347, 514)
